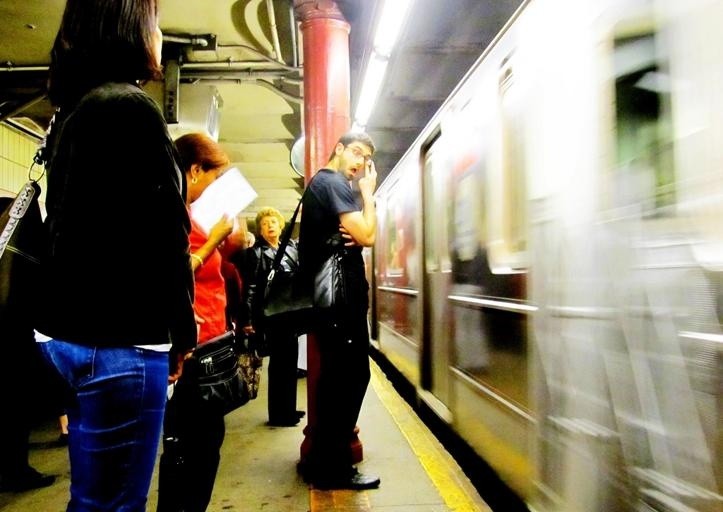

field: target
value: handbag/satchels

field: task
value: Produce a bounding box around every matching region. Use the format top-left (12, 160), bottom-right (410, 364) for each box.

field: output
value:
top-left (179, 331), bottom-right (250, 425)
top-left (259, 252), bottom-right (347, 330)
top-left (0, 179), bottom-right (47, 301)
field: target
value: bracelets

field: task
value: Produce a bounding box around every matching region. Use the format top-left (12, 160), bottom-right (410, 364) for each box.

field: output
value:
top-left (190, 253), bottom-right (204, 269)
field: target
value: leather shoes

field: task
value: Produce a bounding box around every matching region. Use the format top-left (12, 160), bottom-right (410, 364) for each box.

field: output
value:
top-left (0, 466), bottom-right (58, 492)
top-left (349, 471), bottom-right (380, 491)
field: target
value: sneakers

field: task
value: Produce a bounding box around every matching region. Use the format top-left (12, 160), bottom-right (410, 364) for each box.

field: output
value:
top-left (269, 410), bottom-right (306, 428)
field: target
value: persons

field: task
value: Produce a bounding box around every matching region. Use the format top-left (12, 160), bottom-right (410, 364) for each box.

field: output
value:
top-left (389, 210), bottom-right (419, 337)
top-left (48, 409), bottom-right (70, 447)
top-left (22, 2), bottom-right (209, 512)
top-left (294, 126), bottom-right (385, 492)
top-left (242, 206), bottom-right (306, 428)
top-left (445, 180), bottom-right (494, 375)
top-left (154, 132), bottom-right (235, 511)
top-left (0, 193), bottom-right (58, 495)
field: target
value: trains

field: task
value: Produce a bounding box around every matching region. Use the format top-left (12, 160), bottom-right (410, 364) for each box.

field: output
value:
top-left (364, 0), bottom-right (722, 508)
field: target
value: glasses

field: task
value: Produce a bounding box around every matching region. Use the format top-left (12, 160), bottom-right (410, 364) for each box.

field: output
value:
top-left (341, 143), bottom-right (371, 161)
